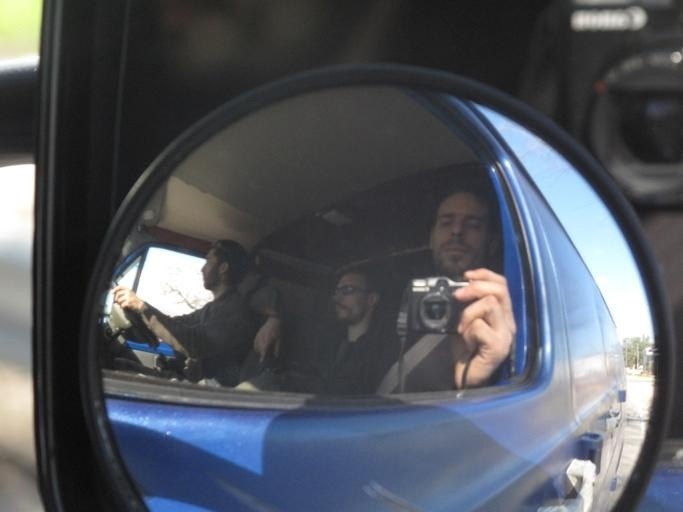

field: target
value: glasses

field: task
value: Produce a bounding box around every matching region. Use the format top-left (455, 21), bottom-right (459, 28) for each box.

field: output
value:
top-left (334, 285), bottom-right (369, 295)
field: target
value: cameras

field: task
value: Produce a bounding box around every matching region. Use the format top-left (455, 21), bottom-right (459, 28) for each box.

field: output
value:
top-left (407, 277), bottom-right (470, 334)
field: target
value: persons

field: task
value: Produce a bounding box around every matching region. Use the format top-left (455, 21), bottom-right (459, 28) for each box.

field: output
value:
top-left (376, 189), bottom-right (516, 394)
top-left (252, 269), bottom-right (398, 394)
top-left (112, 238), bottom-right (254, 386)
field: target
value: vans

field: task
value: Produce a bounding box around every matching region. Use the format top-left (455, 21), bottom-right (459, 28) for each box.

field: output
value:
top-left (98, 88), bottom-right (626, 511)
